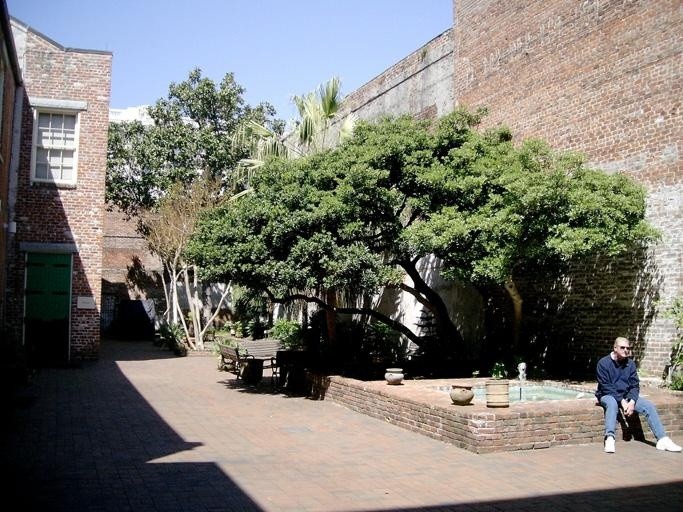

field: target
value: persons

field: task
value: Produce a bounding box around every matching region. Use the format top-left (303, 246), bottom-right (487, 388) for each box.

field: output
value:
top-left (595, 336), bottom-right (683, 453)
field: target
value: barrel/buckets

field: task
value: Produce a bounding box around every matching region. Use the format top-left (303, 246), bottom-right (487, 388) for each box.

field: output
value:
top-left (485, 378), bottom-right (510, 407)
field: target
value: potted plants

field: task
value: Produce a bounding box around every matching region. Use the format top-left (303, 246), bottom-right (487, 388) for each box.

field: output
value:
top-left (224, 320), bottom-right (243, 337)
top-left (207, 330), bottom-right (215, 340)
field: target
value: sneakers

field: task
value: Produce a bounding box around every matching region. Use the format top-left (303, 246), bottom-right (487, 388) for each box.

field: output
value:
top-left (604, 439), bottom-right (615, 453)
top-left (656, 437), bottom-right (682, 452)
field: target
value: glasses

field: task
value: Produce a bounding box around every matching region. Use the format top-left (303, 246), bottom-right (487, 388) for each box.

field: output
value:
top-left (617, 345), bottom-right (630, 350)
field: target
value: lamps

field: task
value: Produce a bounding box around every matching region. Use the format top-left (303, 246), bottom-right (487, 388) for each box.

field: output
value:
top-left (4, 222), bottom-right (17, 233)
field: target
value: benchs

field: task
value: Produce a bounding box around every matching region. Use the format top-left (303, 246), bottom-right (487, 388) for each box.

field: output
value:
top-left (218, 340), bottom-right (307, 393)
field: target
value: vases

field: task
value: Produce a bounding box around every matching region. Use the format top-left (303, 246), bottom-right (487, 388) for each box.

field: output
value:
top-left (385, 367), bottom-right (405, 384)
top-left (450, 385), bottom-right (474, 405)
